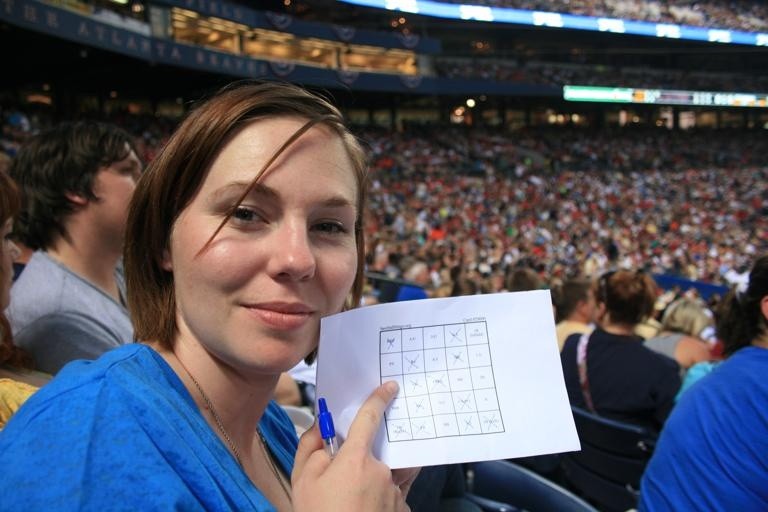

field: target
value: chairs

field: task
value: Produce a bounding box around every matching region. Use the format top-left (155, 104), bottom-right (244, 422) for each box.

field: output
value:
top-left (457, 458), bottom-right (606, 511)
top-left (567, 404), bottom-right (655, 494)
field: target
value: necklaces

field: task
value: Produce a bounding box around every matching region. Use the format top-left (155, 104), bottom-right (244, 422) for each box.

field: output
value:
top-left (173, 351), bottom-right (293, 506)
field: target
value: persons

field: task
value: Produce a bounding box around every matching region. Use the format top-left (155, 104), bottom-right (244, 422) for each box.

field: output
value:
top-left (1, 79), bottom-right (421, 512)
top-left (0, 103), bottom-right (186, 434)
top-left (272, 80), bottom-right (768, 512)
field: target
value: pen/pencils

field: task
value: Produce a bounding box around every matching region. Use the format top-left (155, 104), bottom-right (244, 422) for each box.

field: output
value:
top-left (319, 397), bottom-right (339, 456)
